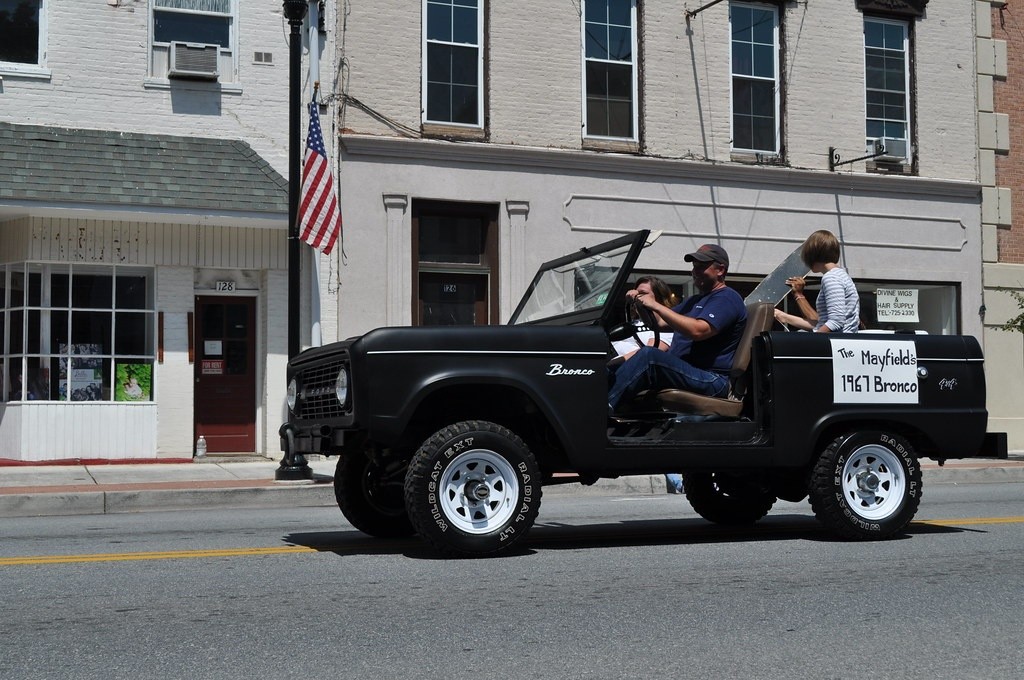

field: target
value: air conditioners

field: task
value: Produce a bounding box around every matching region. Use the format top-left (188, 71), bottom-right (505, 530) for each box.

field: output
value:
top-left (873, 137), bottom-right (907, 161)
top-left (168, 41), bottom-right (220, 81)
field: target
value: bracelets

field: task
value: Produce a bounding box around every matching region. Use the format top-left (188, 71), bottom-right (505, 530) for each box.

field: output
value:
top-left (793, 295), bottom-right (805, 301)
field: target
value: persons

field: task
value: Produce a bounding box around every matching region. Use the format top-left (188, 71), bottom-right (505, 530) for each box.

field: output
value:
top-left (59, 345), bottom-right (103, 400)
top-left (769, 229), bottom-right (860, 332)
top-left (605, 275), bottom-right (678, 363)
top-left (608, 243), bottom-right (747, 415)
top-left (122, 379), bottom-right (142, 397)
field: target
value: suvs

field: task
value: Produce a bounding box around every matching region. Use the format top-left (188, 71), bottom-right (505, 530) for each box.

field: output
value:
top-left (275, 228), bottom-right (991, 557)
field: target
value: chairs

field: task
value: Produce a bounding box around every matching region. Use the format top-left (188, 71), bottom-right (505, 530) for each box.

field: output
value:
top-left (632, 303), bottom-right (774, 417)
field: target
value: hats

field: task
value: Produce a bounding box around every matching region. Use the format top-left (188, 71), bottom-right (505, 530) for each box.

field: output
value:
top-left (684, 244), bottom-right (730, 266)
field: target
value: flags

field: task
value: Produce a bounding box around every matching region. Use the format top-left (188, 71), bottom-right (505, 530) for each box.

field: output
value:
top-left (294, 101), bottom-right (341, 254)
